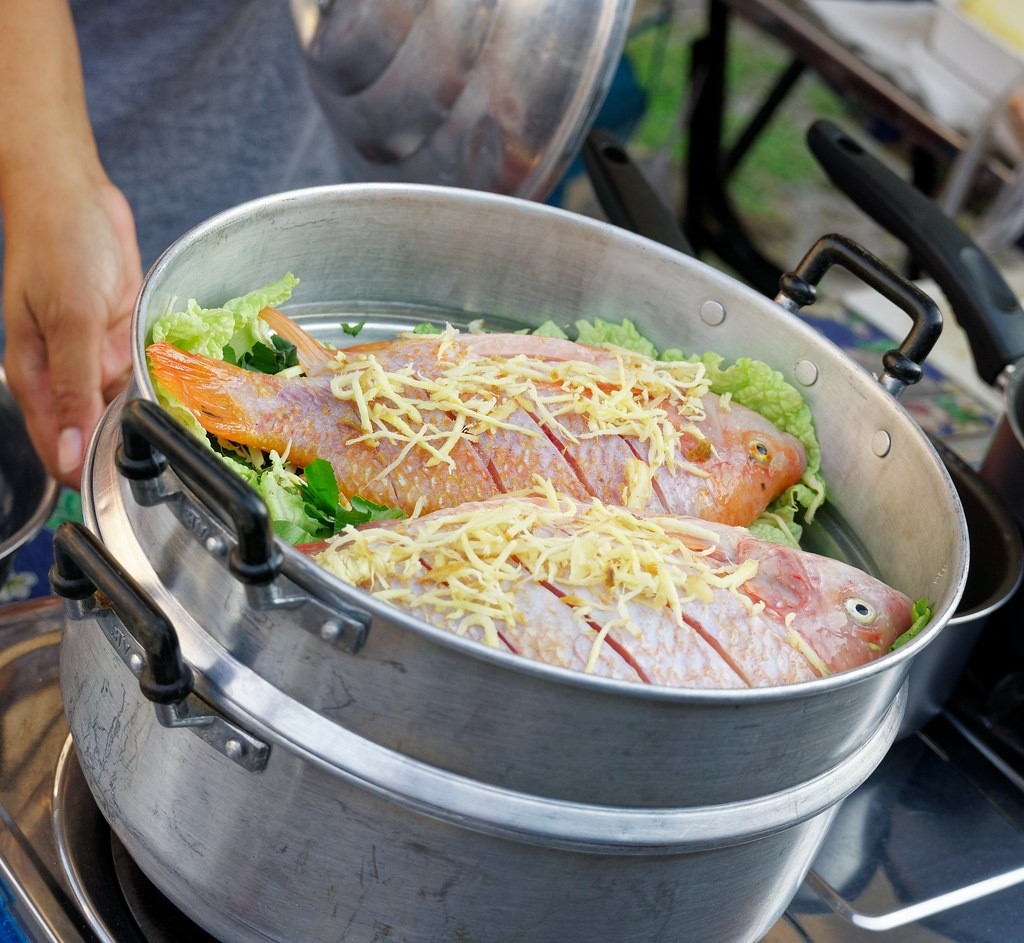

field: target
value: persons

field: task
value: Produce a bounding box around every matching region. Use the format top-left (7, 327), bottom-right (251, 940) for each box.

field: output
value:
top-left (0, 0), bottom-right (345, 498)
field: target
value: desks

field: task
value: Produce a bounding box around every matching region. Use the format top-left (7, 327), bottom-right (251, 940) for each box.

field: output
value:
top-left (680, 0), bottom-right (1024, 308)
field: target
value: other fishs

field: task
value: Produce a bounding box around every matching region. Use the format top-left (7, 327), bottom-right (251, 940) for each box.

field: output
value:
top-left (142, 304), bottom-right (917, 692)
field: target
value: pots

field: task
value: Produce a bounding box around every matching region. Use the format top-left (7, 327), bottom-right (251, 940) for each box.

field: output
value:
top-left (578, 128), bottom-right (1023, 742)
top-left (806, 118), bottom-right (1024, 552)
top-left (54, 183), bottom-right (970, 941)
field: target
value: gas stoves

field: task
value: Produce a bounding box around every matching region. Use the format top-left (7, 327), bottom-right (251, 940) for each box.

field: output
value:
top-left (0, 594), bottom-right (1024, 942)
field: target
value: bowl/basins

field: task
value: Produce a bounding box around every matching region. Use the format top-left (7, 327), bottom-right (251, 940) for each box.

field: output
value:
top-left (1, 365), bottom-right (59, 590)
